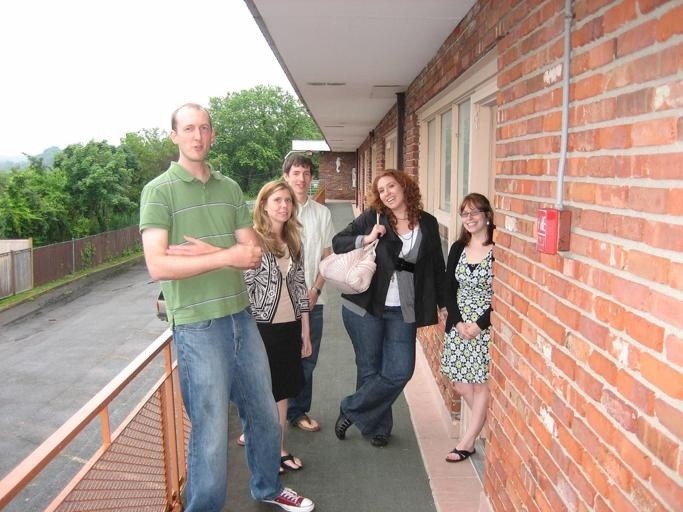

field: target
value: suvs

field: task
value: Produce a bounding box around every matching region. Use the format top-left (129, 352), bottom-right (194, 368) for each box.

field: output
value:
top-left (154, 290), bottom-right (168, 323)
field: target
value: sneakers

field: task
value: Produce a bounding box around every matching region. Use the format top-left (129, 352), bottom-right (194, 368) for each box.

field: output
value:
top-left (334, 397), bottom-right (356, 441)
top-left (249, 481), bottom-right (315, 512)
top-left (369, 437), bottom-right (390, 448)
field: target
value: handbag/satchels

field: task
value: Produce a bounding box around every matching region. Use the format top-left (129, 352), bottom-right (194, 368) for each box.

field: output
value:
top-left (316, 200), bottom-right (384, 297)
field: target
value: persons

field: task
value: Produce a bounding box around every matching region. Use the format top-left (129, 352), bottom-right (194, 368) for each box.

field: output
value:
top-left (139, 103), bottom-right (316, 511)
top-left (446, 192), bottom-right (494, 462)
top-left (237, 179), bottom-right (313, 475)
top-left (331, 168), bottom-right (447, 448)
top-left (237, 154), bottom-right (335, 447)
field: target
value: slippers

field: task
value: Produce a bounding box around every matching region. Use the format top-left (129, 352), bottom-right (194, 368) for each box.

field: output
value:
top-left (445, 446), bottom-right (476, 463)
top-left (236, 433), bottom-right (246, 446)
top-left (281, 450), bottom-right (304, 473)
top-left (292, 413), bottom-right (321, 433)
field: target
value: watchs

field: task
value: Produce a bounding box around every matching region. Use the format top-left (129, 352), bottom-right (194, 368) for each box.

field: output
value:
top-left (312, 286), bottom-right (321, 296)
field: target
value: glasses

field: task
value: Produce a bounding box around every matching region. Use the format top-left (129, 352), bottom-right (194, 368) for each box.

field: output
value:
top-left (459, 208), bottom-right (491, 218)
